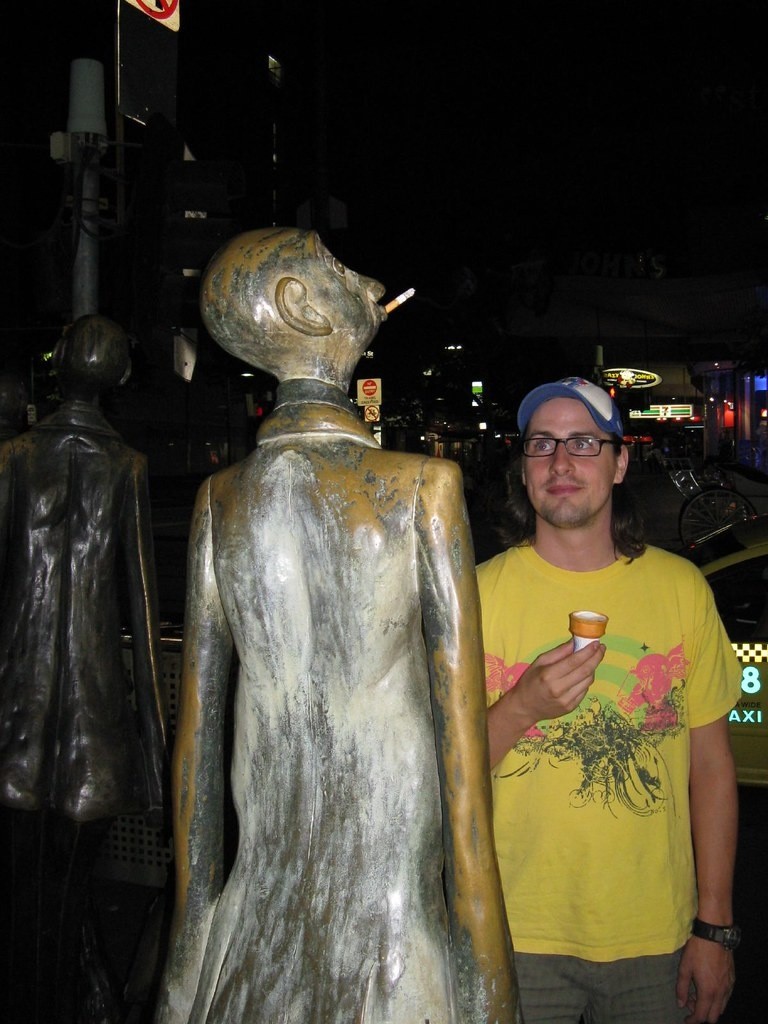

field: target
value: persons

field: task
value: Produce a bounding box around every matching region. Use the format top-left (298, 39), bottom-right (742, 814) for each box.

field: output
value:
top-left (156, 226), bottom-right (515, 1023)
top-left (474, 375), bottom-right (742, 1024)
top-left (0, 315), bottom-right (172, 823)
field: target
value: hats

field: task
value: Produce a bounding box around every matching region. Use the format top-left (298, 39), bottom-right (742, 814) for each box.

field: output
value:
top-left (517, 377), bottom-right (624, 440)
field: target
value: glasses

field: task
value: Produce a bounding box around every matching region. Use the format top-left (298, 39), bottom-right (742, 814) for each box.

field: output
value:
top-left (523, 437), bottom-right (619, 458)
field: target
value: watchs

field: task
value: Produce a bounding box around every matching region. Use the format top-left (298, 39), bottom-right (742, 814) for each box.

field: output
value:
top-left (691, 918), bottom-right (742, 950)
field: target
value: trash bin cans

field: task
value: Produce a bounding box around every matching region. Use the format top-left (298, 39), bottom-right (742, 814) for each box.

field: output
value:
top-left (100, 616), bottom-right (183, 891)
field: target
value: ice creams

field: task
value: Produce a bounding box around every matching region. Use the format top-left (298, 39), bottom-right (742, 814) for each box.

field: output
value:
top-left (568, 612), bottom-right (609, 655)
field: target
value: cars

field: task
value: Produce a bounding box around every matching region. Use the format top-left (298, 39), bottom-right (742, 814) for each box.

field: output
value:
top-left (668, 512), bottom-right (768, 790)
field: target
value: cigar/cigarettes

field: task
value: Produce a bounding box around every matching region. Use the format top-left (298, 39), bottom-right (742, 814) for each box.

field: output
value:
top-left (385, 286), bottom-right (415, 313)
